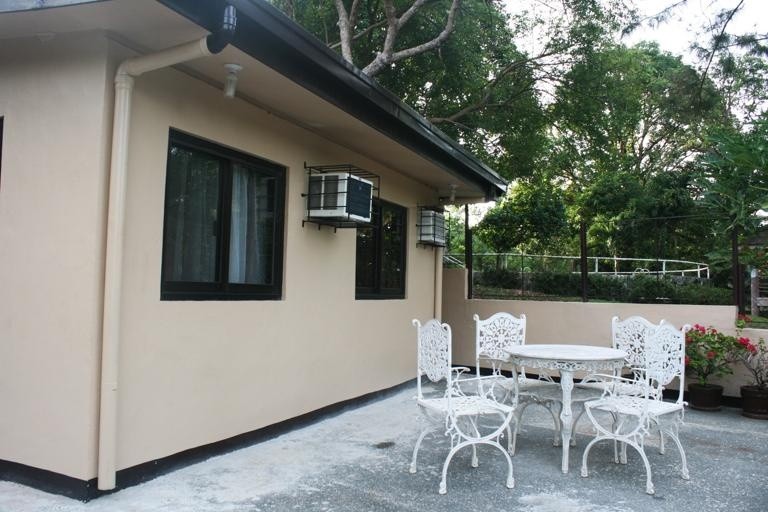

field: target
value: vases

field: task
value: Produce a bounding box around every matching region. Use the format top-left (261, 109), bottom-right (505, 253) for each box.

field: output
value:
top-left (690, 383), bottom-right (768, 415)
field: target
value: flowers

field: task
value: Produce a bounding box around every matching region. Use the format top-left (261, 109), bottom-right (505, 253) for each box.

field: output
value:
top-left (672, 324), bottom-right (767, 381)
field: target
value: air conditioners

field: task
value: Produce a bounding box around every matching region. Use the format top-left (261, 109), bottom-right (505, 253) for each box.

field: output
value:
top-left (419, 210), bottom-right (447, 246)
top-left (306, 171), bottom-right (374, 224)
